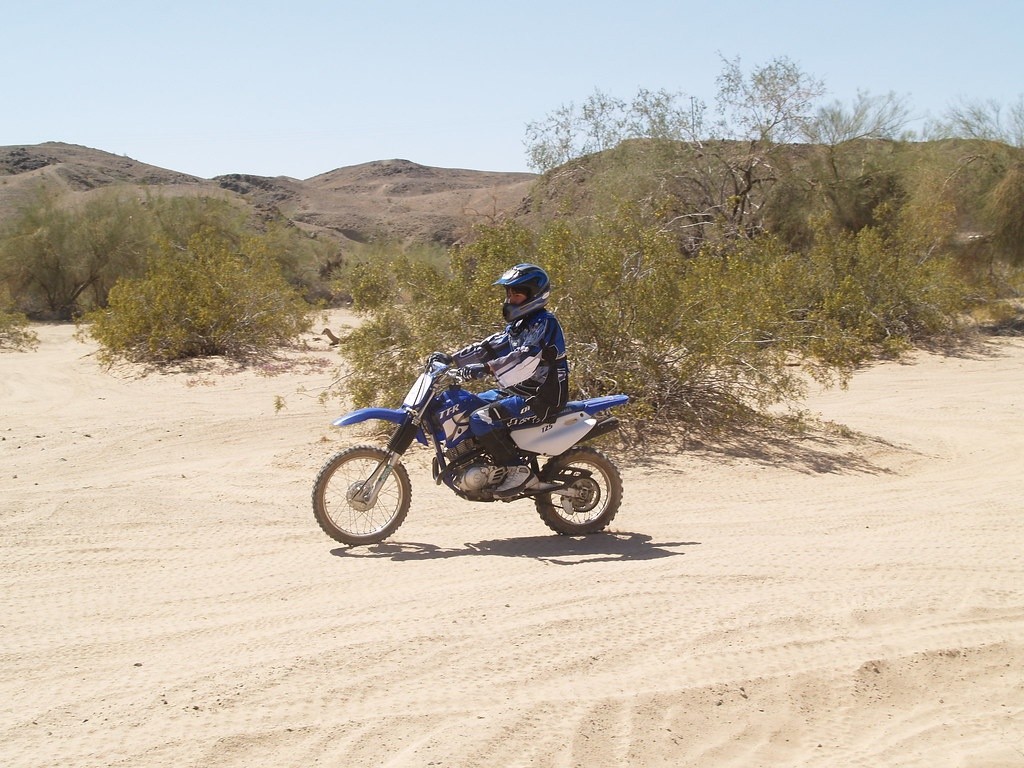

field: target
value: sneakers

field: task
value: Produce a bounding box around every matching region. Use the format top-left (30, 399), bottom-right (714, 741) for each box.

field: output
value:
top-left (492, 464), bottom-right (539, 499)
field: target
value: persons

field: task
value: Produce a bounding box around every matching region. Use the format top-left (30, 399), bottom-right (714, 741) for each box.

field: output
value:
top-left (425, 263), bottom-right (570, 498)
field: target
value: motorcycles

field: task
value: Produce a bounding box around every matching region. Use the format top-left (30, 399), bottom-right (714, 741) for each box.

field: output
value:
top-left (311, 352), bottom-right (633, 546)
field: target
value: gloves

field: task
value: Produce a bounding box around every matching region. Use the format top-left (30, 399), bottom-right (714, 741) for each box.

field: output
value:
top-left (425, 351), bottom-right (453, 366)
top-left (457, 363), bottom-right (485, 381)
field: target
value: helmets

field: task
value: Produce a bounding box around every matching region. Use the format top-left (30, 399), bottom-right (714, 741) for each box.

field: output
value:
top-left (491, 263), bottom-right (551, 322)
